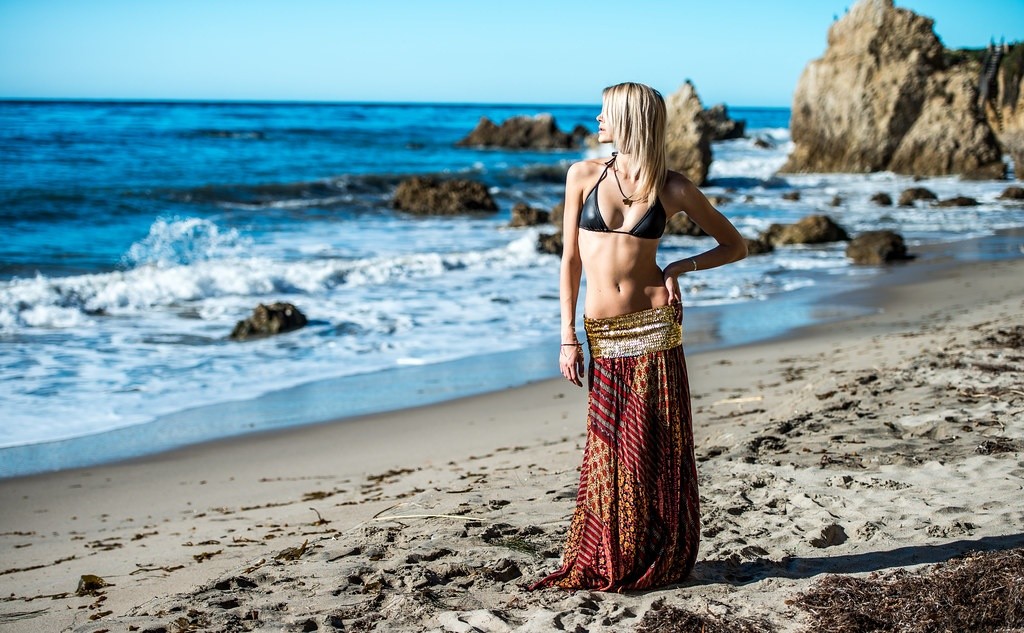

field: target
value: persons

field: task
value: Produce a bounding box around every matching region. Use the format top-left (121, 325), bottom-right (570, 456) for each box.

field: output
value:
top-left (527, 82), bottom-right (748, 594)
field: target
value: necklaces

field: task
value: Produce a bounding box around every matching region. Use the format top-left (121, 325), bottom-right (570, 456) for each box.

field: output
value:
top-left (613, 160), bottom-right (638, 207)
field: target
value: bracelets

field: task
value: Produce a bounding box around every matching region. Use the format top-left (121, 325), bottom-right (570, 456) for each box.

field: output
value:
top-left (688, 258), bottom-right (697, 271)
top-left (560, 343), bottom-right (579, 360)
